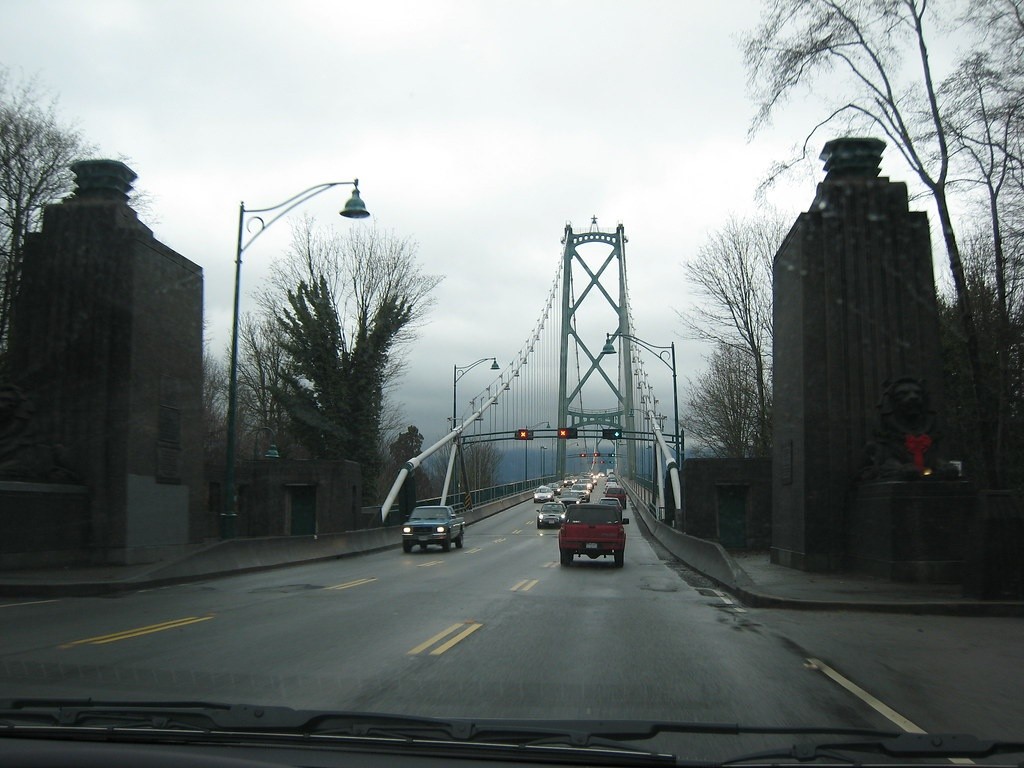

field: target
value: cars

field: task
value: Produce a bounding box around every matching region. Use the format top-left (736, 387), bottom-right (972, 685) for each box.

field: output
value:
top-left (546, 482), bottom-right (562, 496)
top-left (536, 502), bottom-right (566, 528)
top-left (568, 471), bottom-right (618, 502)
top-left (564, 476), bottom-right (576, 487)
top-left (599, 498), bottom-right (623, 522)
top-left (533, 486), bottom-right (555, 503)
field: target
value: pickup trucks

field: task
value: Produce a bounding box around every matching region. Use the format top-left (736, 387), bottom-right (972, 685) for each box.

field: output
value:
top-left (603, 487), bottom-right (628, 509)
top-left (558, 503), bottom-right (630, 567)
top-left (400, 506), bottom-right (465, 550)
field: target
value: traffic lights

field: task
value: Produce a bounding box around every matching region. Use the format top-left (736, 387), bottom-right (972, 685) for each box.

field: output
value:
top-left (611, 453), bottom-right (615, 457)
top-left (580, 454), bottom-right (586, 457)
top-left (518, 428), bottom-right (528, 440)
top-left (558, 429), bottom-right (569, 439)
top-left (594, 453), bottom-right (599, 456)
top-left (610, 427), bottom-right (623, 440)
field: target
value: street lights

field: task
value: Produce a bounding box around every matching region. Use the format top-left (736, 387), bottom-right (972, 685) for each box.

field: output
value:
top-left (218, 177), bottom-right (373, 541)
top-left (453, 357), bottom-right (501, 441)
top-left (600, 330), bottom-right (684, 473)
